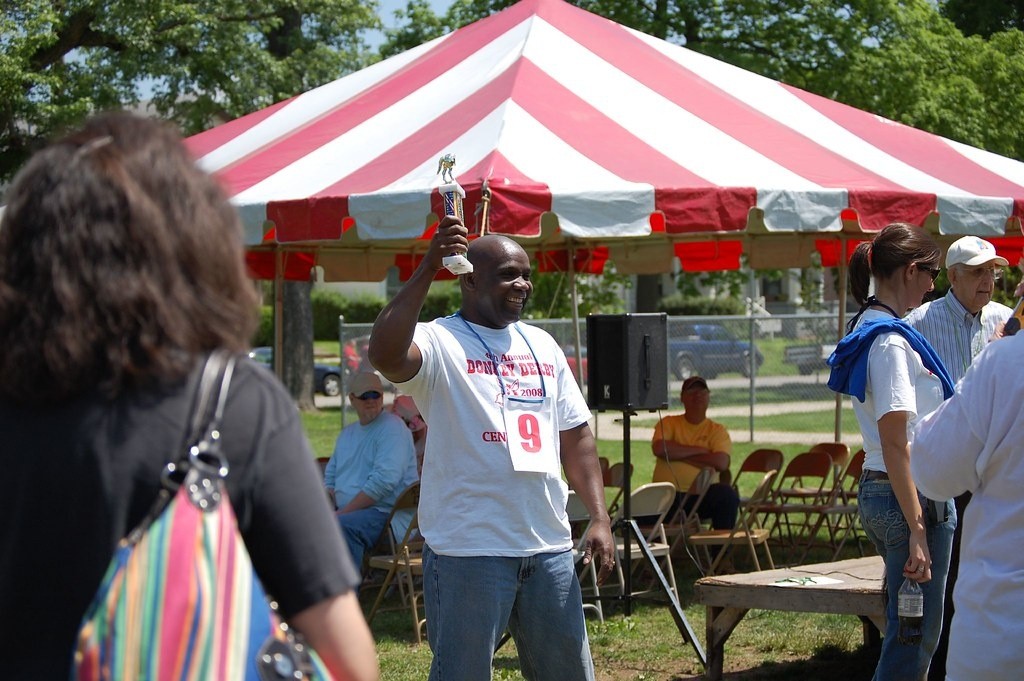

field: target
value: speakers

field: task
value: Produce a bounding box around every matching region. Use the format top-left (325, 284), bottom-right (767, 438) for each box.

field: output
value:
top-left (587, 312), bottom-right (668, 413)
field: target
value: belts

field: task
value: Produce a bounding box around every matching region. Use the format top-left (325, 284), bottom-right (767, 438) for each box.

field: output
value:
top-left (862, 471), bottom-right (889, 480)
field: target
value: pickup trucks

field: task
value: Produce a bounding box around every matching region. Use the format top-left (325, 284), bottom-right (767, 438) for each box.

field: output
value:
top-left (668, 325), bottom-right (765, 381)
top-left (781, 343), bottom-right (838, 375)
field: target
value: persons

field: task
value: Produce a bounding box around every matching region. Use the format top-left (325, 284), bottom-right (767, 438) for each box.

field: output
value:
top-left (324, 372), bottom-right (428, 598)
top-left (0, 108), bottom-right (379, 681)
top-left (827, 223), bottom-right (1024, 681)
top-left (652, 376), bottom-right (739, 574)
top-left (368, 215), bottom-right (614, 681)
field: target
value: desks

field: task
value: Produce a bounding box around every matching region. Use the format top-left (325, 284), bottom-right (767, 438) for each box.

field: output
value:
top-left (693, 553), bottom-right (886, 681)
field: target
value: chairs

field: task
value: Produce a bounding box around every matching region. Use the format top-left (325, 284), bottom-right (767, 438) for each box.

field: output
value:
top-left (570, 481), bottom-right (680, 614)
top-left (630, 465), bottom-right (716, 589)
top-left (599, 457), bottom-right (609, 475)
top-left (797, 448), bottom-right (866, 564)
top-left (690, 471), bottom-right (777, 577)
top-left (708, 449), bottom-right (782, 533)
top-left (761, 451), bottom-right (833, 546)
top-left (366, 511), bottom-right (427, 645)
top-left (564, 489), bottom-right (604, 625)
top-left (769, 443), bottom-right (851, 541)
top-left (357, 480), bottom-right (421, 613)
top-left (605, 463), bottom-right (636, 522)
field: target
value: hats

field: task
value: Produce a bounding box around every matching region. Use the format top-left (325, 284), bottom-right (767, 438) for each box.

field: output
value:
top-left (945, 235), bottom-right (1009, 268)
top-left (349, 373), bottom-right (383, 397)
top-left (684, 376), bottom-right (707, 390)
top-left (393, 396), bottom-right (425, 431)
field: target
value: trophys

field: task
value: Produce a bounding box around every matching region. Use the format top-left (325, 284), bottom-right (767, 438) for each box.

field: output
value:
top-left (436, 154), bottom-right (473, 275)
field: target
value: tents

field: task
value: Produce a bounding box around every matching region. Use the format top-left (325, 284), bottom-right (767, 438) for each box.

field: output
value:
top-left (182, 0), bottom-right (1024, 536)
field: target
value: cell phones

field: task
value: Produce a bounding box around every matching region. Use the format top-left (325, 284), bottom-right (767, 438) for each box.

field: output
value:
top-left (1003, 296), bottom-right (1024, 337)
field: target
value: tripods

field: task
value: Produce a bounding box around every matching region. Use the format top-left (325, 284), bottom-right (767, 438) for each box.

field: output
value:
top-left (494, 410), bottom-right (707, 670)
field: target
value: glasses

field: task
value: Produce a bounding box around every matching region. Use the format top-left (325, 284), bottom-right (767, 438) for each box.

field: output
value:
top-left (965, 268), bottom-right (1005, 279)
top-left (353, 392), bottom-right (380, 400)
top-left (916, 266), bottom-right (942, 282)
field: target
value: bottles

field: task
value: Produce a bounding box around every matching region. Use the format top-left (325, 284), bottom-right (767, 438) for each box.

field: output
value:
top-left (897, 571), bottom-right (924, 645)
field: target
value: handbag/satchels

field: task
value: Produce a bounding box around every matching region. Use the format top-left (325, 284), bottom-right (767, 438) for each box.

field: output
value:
top-left (70, 348), bottom-right (336, 681)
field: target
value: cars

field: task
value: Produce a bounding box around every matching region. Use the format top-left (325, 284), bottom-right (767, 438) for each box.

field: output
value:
top-left (245, 346), bottom-right (341, 397)
top-left (560, 346), bottom-right (587, 386)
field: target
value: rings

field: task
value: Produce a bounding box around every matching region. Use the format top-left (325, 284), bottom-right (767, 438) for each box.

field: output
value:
top-left (918, 565), bottom-right (924, 572)
top-left (613, 561), bottom-right (615, 565)
